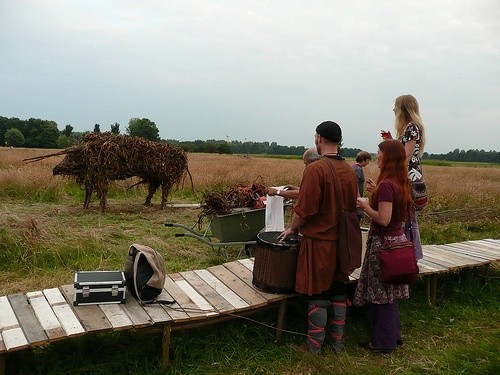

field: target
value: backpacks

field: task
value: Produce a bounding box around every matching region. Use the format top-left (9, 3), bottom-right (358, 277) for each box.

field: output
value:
top-left (123, 243), bottom-right (166, 305)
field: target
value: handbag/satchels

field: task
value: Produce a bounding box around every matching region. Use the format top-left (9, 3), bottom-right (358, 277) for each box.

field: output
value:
top-left (378, 240), bottom-right (420, 285)
top-left (410, 179), bottom-right (427, 211)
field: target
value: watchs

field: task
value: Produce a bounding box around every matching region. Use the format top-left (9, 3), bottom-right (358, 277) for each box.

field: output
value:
top-left (277, 190), bottom-right (281, 195)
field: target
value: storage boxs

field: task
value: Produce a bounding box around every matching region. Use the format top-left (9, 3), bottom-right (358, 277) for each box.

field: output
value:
top-left (72, 271), bottom-right (127, 306)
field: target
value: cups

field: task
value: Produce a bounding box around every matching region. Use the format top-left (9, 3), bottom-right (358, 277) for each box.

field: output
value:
top-left (382, 130), bottom-right (388, 139)
top-left (357, 197), bottom-right (367, 211)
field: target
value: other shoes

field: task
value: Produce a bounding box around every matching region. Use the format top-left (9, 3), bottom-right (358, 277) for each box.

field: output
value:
top-left (360, 342), bottom-right (393, 356)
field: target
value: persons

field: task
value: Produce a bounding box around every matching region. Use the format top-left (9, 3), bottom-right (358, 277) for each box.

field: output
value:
top-left (351, 151), bottom-right (371, 228)
top-left (356, 140), bottom-right (419, 353)
top-left (380, 95), bottom-right (424, 259)
top-left (357, 177), bottom-right (422, 260)
top-left (264, 148), bottom-right (321, 199)
top-left (277, 121), bottom-right (358, 352)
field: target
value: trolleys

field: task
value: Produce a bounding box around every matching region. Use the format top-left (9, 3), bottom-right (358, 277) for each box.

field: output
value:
top-left (164, 196), bottom-right (294, 261)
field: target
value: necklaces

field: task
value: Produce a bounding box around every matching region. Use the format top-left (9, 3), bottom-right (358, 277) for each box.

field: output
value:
top-left (323, 152), bottom-right (340, 155)
